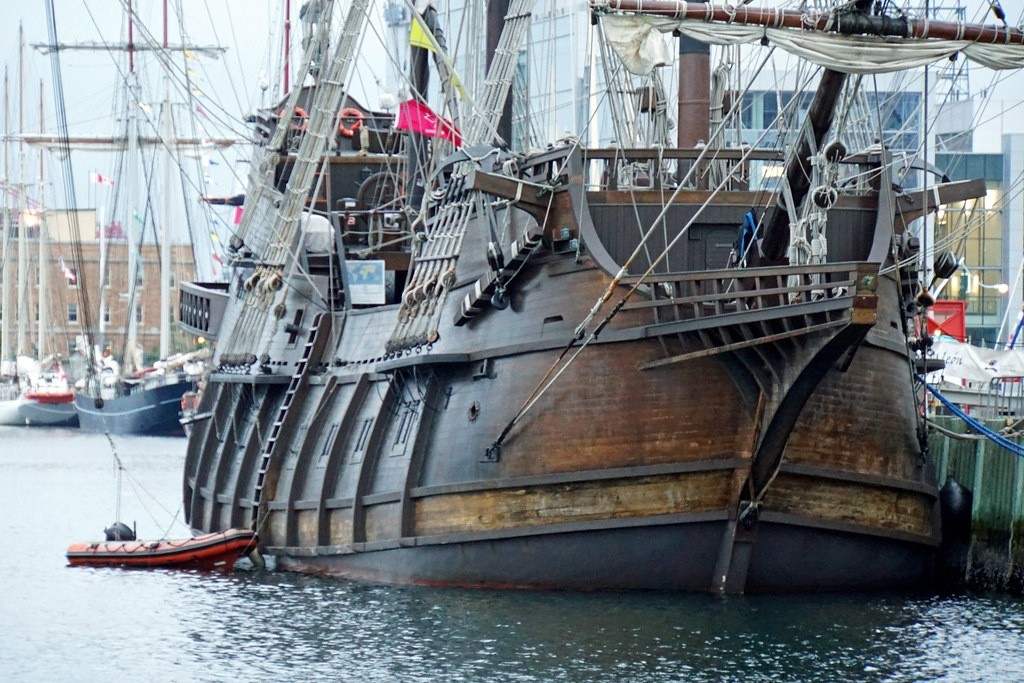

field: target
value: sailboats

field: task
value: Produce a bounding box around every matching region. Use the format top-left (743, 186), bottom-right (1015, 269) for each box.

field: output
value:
top-left (0, 1), bottom-right (1024, 606)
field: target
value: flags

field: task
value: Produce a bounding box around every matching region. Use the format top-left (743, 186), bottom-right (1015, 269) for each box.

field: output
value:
top-left (95, 173), bottom-right (114, 186)
top-left (394, 98), bottom-right (462, 147)
top-left (60, 257), bottom-right (75, 283)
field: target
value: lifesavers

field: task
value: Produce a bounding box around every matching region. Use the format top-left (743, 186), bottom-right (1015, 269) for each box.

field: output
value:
top-left (335, 108), bottom-right (364, 138)
top-left (279, 106), bottom-right (310, 132)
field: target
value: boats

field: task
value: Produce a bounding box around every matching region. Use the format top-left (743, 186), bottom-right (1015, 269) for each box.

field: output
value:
top-left (64, 521), bottom-right (259, 575)
